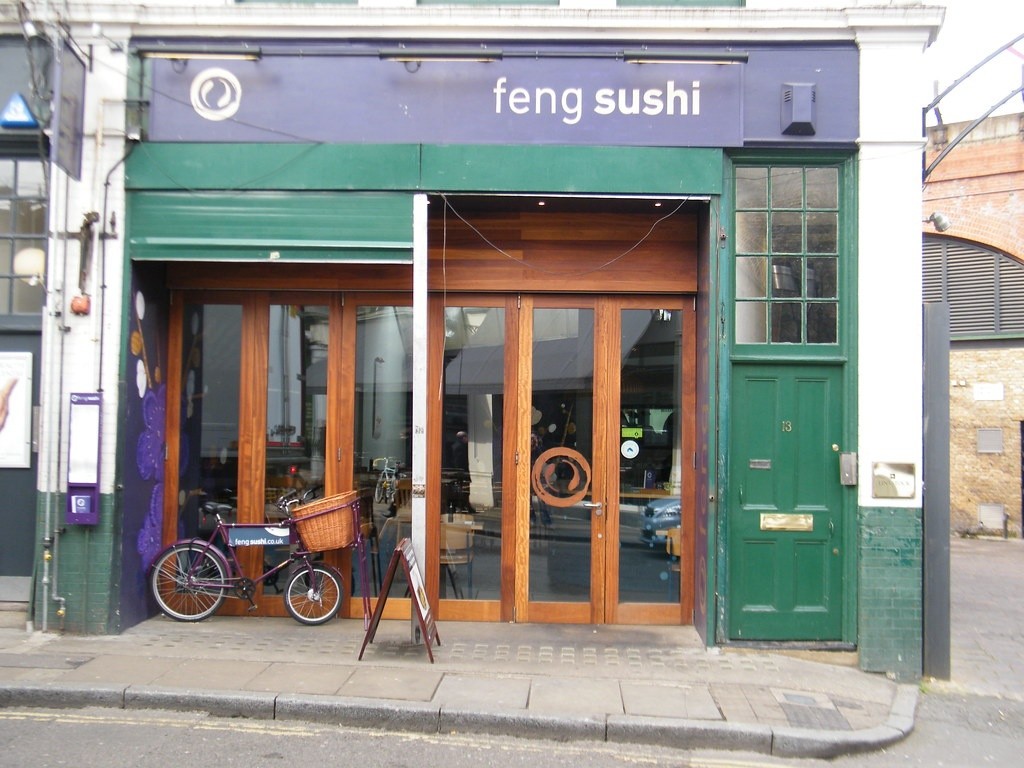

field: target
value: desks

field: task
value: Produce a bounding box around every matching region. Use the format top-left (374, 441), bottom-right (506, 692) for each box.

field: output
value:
top-left (391, 518), bottom-right (486, 599)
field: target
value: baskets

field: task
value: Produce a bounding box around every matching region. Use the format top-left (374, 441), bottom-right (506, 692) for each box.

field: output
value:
top-left (291, 491), bottom-right (357, 552)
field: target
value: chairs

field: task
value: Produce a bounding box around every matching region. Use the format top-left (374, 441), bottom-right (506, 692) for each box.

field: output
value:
top-left (439, 514), bottom-right (474, 601)
top-left (666, 528), bottom-right (681, 603)
top-left (352, 477), bottom-right (411, 597)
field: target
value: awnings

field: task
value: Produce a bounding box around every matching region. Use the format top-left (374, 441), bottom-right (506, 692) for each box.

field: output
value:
top-left (302, 318), bottom-right (414, 393)
top-left (444, 306), bottom-right (658, 395)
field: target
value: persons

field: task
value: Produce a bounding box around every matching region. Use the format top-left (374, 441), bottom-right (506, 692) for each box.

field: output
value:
top-left (530, 425), bottom-right (556, 530)
top-left (451, 431), bottom-right (470, 513)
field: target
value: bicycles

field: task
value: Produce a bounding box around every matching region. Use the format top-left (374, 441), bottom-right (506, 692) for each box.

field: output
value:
top-left (146, 488), bottom-right (345, 624)
top-left (373, 456), bottom-right (406, 503)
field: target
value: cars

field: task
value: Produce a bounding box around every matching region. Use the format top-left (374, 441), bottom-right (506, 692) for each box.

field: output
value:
top-left (641, 493), bottom-right (680, 544)
